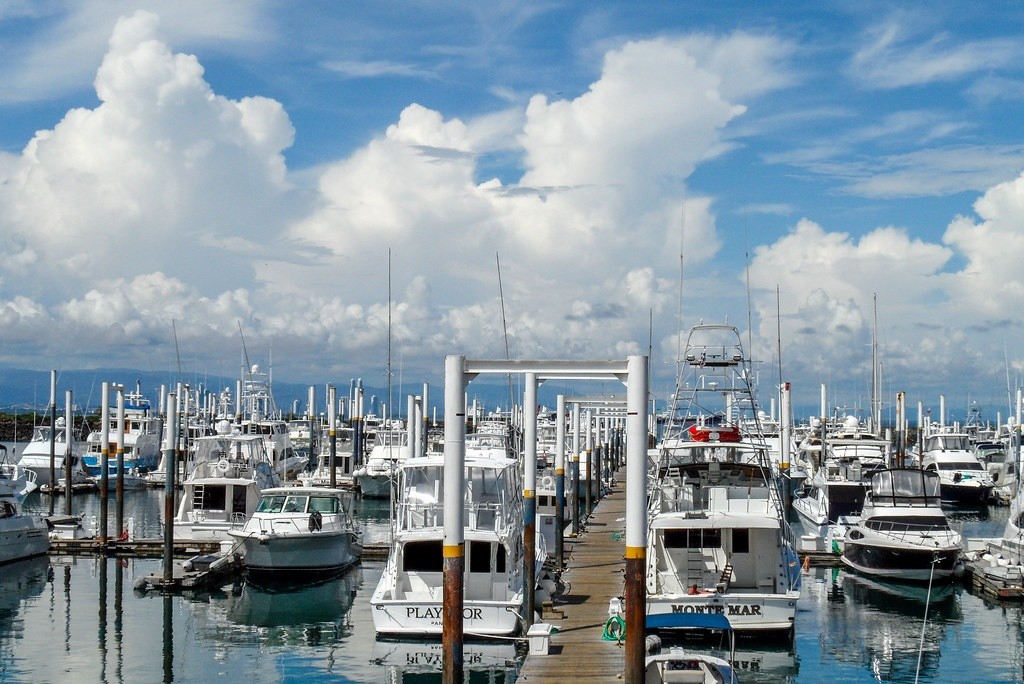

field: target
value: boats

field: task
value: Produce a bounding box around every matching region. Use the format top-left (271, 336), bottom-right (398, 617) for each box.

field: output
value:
top-left (1, 242), bottom-right (1024, 684)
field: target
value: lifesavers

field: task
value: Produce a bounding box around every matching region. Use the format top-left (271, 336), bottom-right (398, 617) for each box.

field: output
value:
top-left (542, 476), bottom-right (553, 488)
top-left (217, 460), bottom-right (230, 473)
